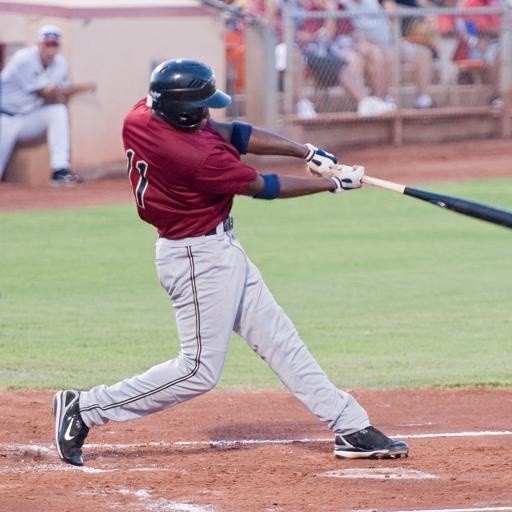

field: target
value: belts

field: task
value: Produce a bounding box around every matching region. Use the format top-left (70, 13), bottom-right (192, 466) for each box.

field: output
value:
top-left (208, 216), bottom-right (234, 235)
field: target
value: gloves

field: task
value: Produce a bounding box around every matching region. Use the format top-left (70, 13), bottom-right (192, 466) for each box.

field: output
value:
top-left (302, 143), bottom-right (338, 175)
top-left (328, 165), bottom-right (365, 192)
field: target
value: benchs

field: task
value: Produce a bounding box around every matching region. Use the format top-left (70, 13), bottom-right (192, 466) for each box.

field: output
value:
top-left (223, 3), bottom-right (501, 128)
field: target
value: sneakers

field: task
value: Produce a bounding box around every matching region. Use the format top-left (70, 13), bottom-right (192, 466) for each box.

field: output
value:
top-left (53, 390), bottom-right (88, 465)
top-left (417, 94), bottom-right (432, 109)
top-left (358, 96), bottom-right (394, 113)
top-left (52, 169), bottom-right (82, 183)
top-left (297, 98), bottom-right (317, 119)
top-left (333, 427), bottom-right (409, 458)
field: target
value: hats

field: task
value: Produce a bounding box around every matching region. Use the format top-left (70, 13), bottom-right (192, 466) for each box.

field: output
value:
top-left (38, 24), bottom-right (61, 47)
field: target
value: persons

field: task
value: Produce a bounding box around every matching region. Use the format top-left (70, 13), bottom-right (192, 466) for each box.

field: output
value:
top-left (54, 60), bottom-right (408, 465)
top-left (218, 0), bottom-right (512, 118)
top-left (0, 26), bottom-right (96, 186)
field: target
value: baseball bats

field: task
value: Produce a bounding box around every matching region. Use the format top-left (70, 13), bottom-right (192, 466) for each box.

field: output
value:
top-left (335, 165), bottom-right (512, 229)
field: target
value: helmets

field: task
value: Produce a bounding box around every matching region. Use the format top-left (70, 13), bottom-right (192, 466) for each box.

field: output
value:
top-left (146, 59), bottom-right (232, 130)
